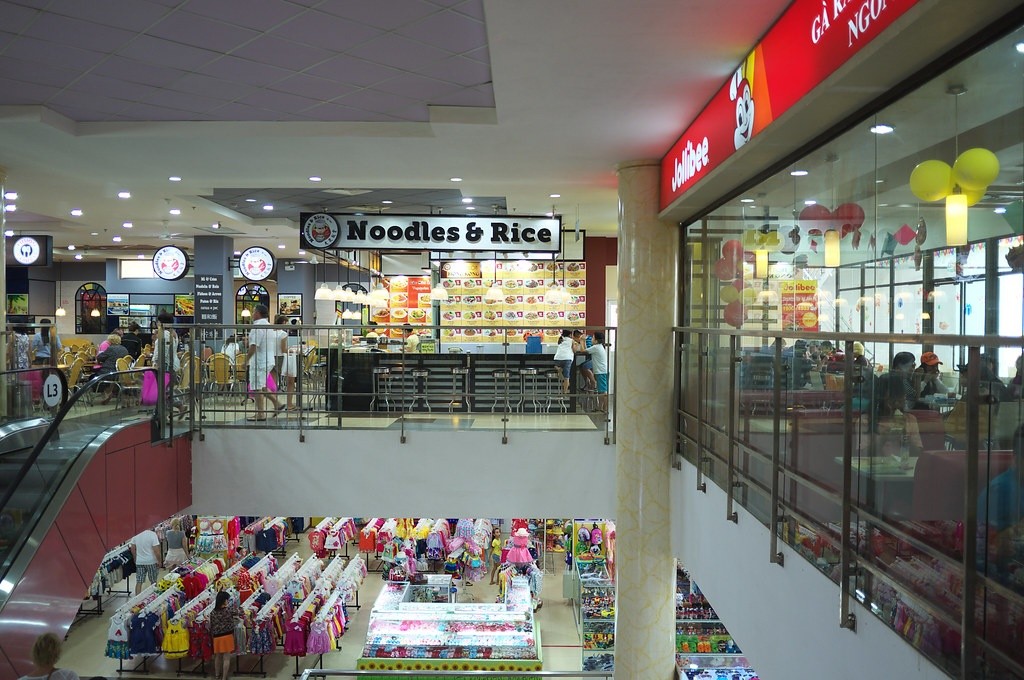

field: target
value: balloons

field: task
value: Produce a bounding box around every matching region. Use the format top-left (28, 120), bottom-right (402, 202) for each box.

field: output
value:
top-left (716, 147), bottom-right (1000, 328)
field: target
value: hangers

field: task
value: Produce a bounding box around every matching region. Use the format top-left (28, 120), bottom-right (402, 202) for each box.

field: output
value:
top-left (98, 517), bottom-right (543, 625)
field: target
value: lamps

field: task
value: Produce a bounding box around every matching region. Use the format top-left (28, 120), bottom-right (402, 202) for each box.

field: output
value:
top-left (753, 248), bottom-right (772, 282)
top-left (487, 251), bottom-right (503, 299)
top-left (314, 249), bottom-right (391, 319)
top-left (545, 227), bottom-right (573, 304)
top-left (823, 155), bottom-right (844, 267)
top-left (430, 250), bottom-right (449, 300)
top-left (56, 259), bottom-right (66, 316)
top-left (241, 300), bottom-right (252, 318)
top-left (90, 283), bottom-right (101, 317)
top-left (945, 85), bottom-right (969, 244)
top-left (213, 221), bottom-right (221, 228)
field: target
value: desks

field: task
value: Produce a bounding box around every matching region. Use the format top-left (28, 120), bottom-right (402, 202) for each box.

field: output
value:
top-left (831, 453), bottom-right (918, 518)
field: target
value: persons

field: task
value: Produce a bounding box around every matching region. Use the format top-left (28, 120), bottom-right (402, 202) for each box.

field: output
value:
top-left (131, 525), bottom-right (162, 597)
top-left (10, 305), bottom-right (609, 422)
top-left (16, 631), bottom-right (81, 680)
top-left (163, 518), bottom-right (190, 573)
top-left (771, 338), bottom-right (1024, 458)
top-left (210, 591), bottom-right (245, 680)
top-left (488, 528), bottom-right (502, 585)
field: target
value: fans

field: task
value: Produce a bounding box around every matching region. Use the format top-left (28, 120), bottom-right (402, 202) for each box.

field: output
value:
top-left (294, 253), bottom-right (334, 265)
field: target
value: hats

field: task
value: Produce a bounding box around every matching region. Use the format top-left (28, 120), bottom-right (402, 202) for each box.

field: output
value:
top-left (956, 362), bottom-right (969, 370)
top-left (921, 351), bottom-right (944, 366)
top-left (807, 340), bottom-right (822, 347)
top-left (515, 528), bottom-right (529, 537)
top-left (853, 343), bottom-right (864, 356)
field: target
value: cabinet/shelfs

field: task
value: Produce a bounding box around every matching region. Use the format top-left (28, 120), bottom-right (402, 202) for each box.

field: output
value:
top-left (575, 558), bottom-right (615, 670)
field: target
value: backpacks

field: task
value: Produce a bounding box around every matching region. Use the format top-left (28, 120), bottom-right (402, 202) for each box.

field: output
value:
top-left (577, 525), bottom-right (603, 545)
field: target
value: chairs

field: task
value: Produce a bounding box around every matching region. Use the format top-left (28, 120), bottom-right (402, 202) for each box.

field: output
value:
top-left (32, 343), bottom-right (325, 423)
top-left (824, 373), bottom-right (843, 414)
top-left (809, 371), bottom-right (825, 391)
top-left (945, 400), bottom-right (995, 453)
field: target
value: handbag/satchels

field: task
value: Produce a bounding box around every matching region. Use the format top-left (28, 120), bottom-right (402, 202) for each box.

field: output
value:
top-left (282, 349), bottom-right (297, 377)
top-left (17, 370), bottom-right (43, 396)
top-left (141, 370), bottom-right (170, 405)
top-left (248, 373), bottom-right (276, 398)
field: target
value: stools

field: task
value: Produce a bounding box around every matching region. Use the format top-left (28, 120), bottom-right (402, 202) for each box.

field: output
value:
top-left (448, 367), bottom-right (472, 414)
top-left (409, 368), bottom-right (433, 414)
top-left (492, 369), bottom-right (513, 415)
top-left (576, 365), bottom-right (600, 412)
top-left (310, 363), bottom-right (325, 409)
top-left (517, 367), bottom-right (542, 413)
top-left (369, 367), bottom-right (396, 414)
top-left (543, 370), bottom-right (568, 413)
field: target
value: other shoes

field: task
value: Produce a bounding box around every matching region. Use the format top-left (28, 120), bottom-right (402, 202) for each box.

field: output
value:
top-left (274, 404), bottom-right (286, 418)
top-left (179, 406), bottom-right (188, 420)
top-left (246, 417), bottom-right (267, 421)
top-left (288, 406), bottom-right (301, 412)
top-left (215, 673), bottom-right (223, 680)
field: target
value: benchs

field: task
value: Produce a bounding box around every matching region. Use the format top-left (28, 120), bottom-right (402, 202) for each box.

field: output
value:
top-left (741, 389), bottom-right (1017, 517)
